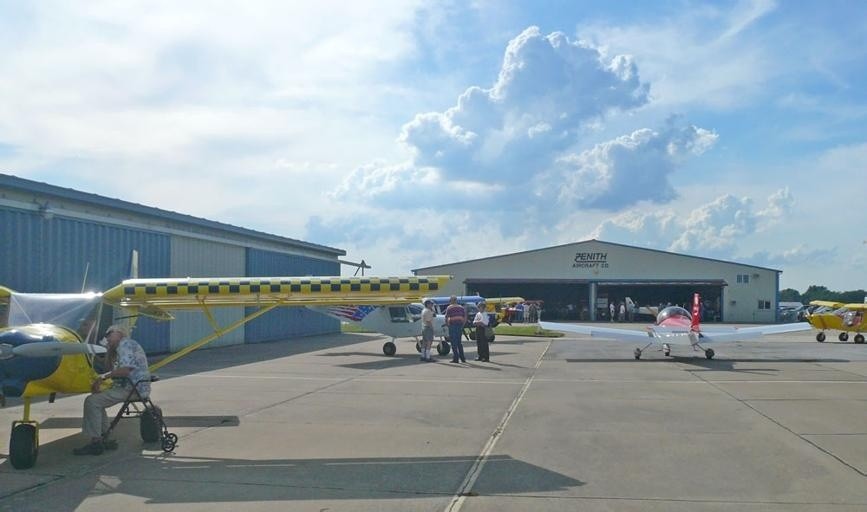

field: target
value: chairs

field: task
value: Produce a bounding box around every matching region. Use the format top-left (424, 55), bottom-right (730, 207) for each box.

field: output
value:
top-left (100, 375), bottom-right (177, 456)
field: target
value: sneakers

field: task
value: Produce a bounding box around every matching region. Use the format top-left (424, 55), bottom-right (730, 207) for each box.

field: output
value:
top-left (73, 438), bottom-right (105, 456)
top-left (101, 437), bottom-right (120, 450)
top-left (421, 357), bottom-right (489, 363)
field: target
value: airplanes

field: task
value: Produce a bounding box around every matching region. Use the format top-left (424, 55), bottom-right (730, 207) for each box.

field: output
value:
top-left (310, 261), bottom-right (458, 357)
top-left (806, 300), bottom-right (867, 345)
top-left (540, 302), bottom-right (812, 365)
top-left (416, 293), bottom-right (501, 343)
top-left (0, 250), bottom-right (454, 470)
top-left (482, 297), bottom-right (527, 320)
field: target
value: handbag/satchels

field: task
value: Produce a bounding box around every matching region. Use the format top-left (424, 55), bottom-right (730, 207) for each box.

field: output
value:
top-left (483, 327), bottom-right (495, 343)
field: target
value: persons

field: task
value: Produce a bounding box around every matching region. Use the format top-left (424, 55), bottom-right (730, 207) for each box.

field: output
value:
top-left (552, 299), bottom-right (708, 323)
top-left (472, 302), bottom-right (489, 362)
top-left (444, 295), bottom-right (466, 364)
top-left (419, 300), bottom-right (436, 363)
top-left (495, 300), bottom-right (541, 323)
top-left (807, 305), bottom-right (814, 316)
top-left (72, 325), bottom-right (151, 455)
